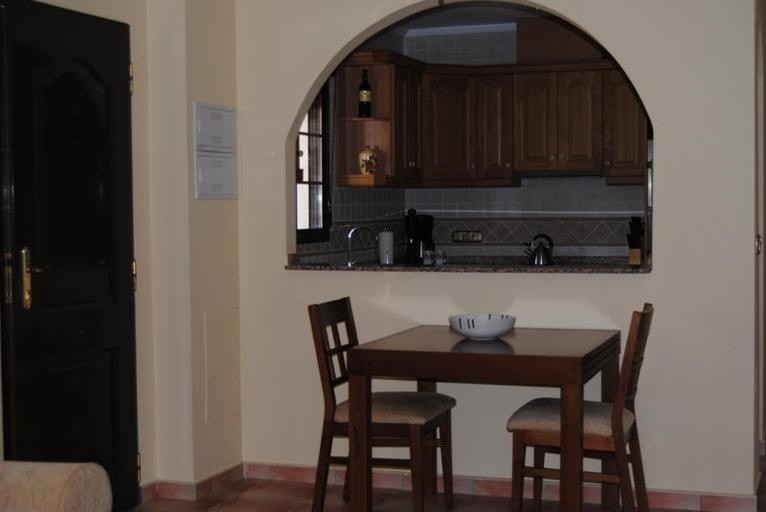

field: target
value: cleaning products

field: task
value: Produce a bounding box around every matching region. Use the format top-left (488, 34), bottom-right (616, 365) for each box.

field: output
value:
top-left (379, 232), bottom-right (395, 266)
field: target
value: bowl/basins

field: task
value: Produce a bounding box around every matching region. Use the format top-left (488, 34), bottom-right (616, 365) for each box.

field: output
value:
top-left (447, 311), bottom-right (517, 342)
top-left (449, 338), bottom-right (517, 356)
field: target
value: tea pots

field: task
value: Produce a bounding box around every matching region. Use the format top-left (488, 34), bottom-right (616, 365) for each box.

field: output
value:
top-left (522, 233), bottom-right (556, 265)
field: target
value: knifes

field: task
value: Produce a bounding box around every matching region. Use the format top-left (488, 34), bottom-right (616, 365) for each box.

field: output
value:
top-left (626, 216), bottom-right (644, 265)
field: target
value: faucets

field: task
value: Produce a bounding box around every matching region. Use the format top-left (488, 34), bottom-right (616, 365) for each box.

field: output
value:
top-left (347, 226), bottom-right (379, 266)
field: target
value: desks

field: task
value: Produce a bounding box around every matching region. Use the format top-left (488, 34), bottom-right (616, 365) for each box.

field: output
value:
top-left (347, 326), bottom-right (621, 512)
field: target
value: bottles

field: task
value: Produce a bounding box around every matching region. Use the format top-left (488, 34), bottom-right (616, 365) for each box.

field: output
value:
top-left (356, 67), bottom-right (373, 118)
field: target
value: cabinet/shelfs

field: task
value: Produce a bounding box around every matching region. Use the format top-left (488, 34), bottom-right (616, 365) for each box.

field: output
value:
top-left (336, 60), bottom-right (420, 187)
top-left (423, 71), bottom-right (599, 187)
top-left (601, 67), bottom-right (650, 185)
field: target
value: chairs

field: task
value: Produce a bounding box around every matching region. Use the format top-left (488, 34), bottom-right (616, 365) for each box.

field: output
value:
top-left (507, 302), bottom-right (654, 512)
top-left (307, 294), bottom-right (458, 510)
